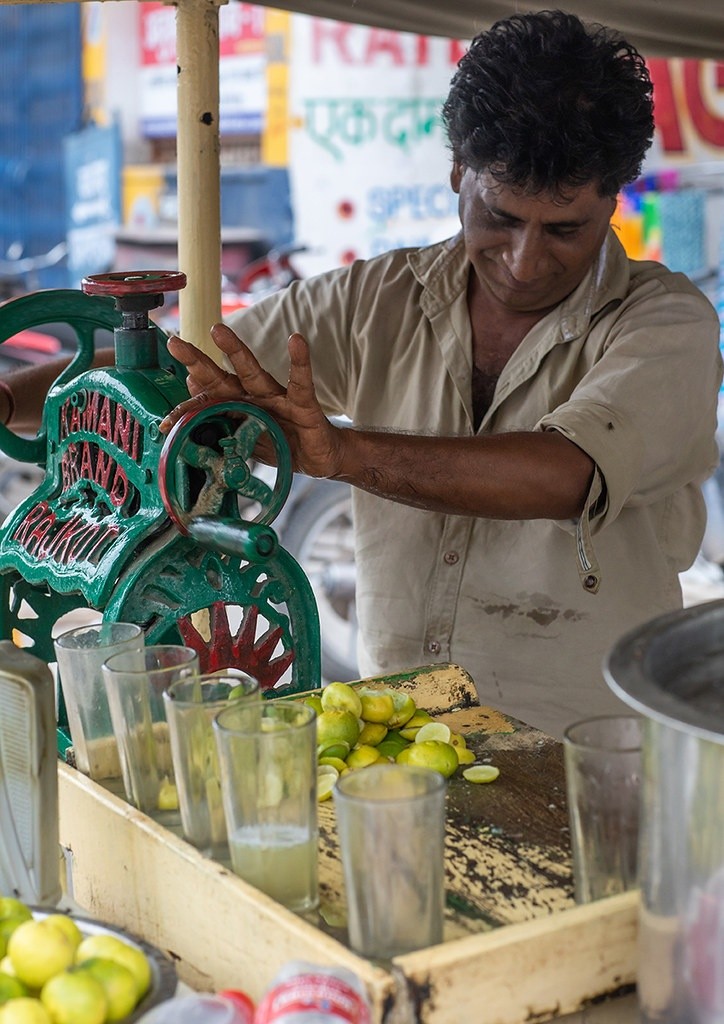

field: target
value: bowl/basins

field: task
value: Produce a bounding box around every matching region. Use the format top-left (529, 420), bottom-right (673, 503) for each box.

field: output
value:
top-left (23, 904), bottom-right (179, 1024)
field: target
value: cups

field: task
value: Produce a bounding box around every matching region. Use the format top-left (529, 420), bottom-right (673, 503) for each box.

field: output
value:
top-left (562, 715), bottom-right (642, 904)
top-left (332, 765), bottom-right (445, 961)
top-left (54, 623), bottom-right (321, 914)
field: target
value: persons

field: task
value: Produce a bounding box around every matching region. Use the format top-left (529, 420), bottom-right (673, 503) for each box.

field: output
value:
top-left (0, 10), bottom-right (724, 760)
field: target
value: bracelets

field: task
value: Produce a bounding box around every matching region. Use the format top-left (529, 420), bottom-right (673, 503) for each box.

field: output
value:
top-left (0, 382), bottom-right (16, 427)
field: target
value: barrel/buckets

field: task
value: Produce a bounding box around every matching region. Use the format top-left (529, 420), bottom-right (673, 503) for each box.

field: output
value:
top-left (604, 599), bottom-right (723, 1024)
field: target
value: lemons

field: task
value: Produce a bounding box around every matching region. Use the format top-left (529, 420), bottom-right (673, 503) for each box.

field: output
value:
top-left (0, 894), bottom-right (150, 1024)
top-left (150, 683), bottom-right (501, 804)
